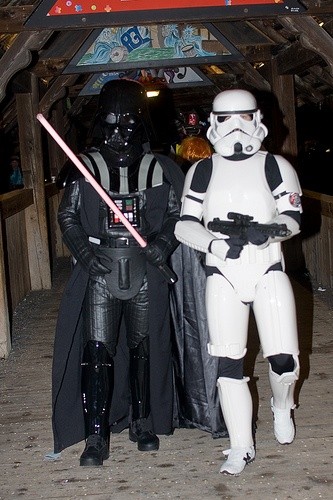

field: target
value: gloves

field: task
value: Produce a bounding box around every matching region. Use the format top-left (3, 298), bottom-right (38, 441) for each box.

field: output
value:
top-left (242, 225), bottom-right (268, 246)
top-left (141, 216), bottom-right (182, 266)
top-left (210, 237), bottom-right (247, 259)
top-left (59, 225), bottom-right (112, 277)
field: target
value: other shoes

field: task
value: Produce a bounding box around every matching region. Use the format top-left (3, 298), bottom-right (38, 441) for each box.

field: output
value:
top-left (270, 395), bottom-right (297, 445)
top-left (219, 446), bottom-right (257, 475)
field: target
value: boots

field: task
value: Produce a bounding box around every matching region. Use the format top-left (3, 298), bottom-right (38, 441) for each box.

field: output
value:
top-left (129, 334), bottom-right (160, 452)
top-left (79, 340), bottom-right (115, 468)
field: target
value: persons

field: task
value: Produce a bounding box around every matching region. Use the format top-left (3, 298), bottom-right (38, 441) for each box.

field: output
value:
top-left (172, 90), bottom-right (305, 476)
top-left (53, 79), bottom-right (228, 466)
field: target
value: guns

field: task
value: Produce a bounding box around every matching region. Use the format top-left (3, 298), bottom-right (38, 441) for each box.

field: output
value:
top-left (208, 212), bottom-right (293, 241)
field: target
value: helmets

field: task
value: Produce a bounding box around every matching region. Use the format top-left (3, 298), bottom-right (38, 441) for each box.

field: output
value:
top-left (205, 89), bottom-right (269, 160)
top-left (94, 78), bottom-right (153, 168)
